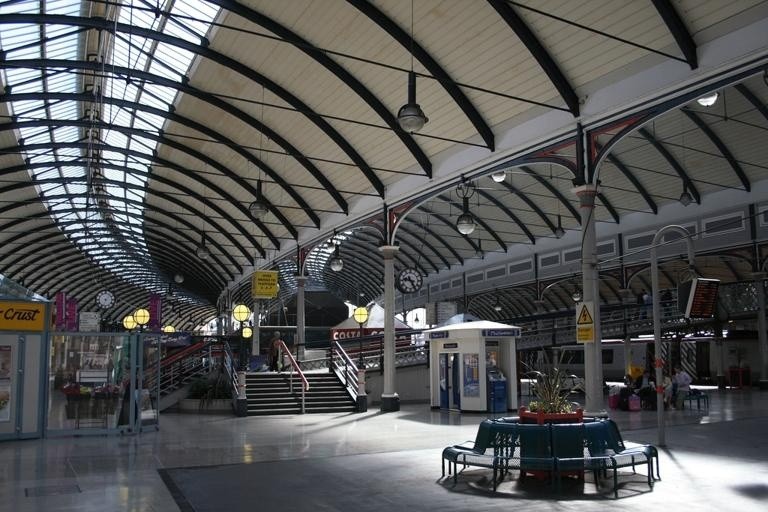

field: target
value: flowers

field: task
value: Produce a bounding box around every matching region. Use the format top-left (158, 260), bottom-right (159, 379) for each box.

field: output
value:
top-left (59, 382), bottom-right (124, 397)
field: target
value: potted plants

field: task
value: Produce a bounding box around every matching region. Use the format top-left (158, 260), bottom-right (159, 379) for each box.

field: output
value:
top-left (519, 347), bottom-right (585, 484)
top-left (724, 346), bottom-right (753, 390)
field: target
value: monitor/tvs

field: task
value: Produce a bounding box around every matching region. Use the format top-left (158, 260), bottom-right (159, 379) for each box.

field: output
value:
top-left (678, 278), bottom-right (721, 318)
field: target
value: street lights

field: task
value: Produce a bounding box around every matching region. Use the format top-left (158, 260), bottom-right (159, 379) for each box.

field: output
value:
top-left (121, 315), bottom-right (137, 333)
top-left (233, 300), bottom-right (252, 370)
top-left (352, 305), bottom-right (372, 370)
top-left (133, 308), bottom-right (151, 332)
top-left (242, 327), bottom-right (251, 371)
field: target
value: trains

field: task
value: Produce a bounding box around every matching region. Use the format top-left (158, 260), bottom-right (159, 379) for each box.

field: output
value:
top-left (549, 335), bottom-right (767, 390)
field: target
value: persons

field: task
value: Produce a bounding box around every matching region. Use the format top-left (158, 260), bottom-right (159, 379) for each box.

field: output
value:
top-left (267, 330), bottom-right (286, 372)
top-left (633, 370), bottom-right (650, 408)
top-left (664, 375), bottom-right (675, 410)
top-left (672, 363), bottom-right (692, 409)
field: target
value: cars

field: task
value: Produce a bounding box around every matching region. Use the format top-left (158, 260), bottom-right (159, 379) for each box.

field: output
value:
top-left (519, 369), bottom-right (583, 394)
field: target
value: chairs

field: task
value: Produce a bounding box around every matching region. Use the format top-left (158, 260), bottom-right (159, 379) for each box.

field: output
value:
top-left (442, 416), bottom-right (661, 499)
top-left (641, 392), bottom-right (709, 411)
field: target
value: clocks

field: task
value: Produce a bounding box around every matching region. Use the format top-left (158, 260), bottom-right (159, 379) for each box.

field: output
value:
top-left (396, 267), bottom-right (423, 294)
top-left (95, 290), bottom-right (115, 310)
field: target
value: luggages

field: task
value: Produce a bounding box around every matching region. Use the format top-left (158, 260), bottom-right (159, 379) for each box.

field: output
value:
top-left (608, 385), bottom-right (657, 411)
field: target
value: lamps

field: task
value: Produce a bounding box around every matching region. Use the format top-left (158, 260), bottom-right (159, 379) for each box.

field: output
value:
top-left (122, 308), bottom-right (150, 333)
top-left (572, 285), bottom-right (584, 304)
top-left (697, 92), bottom-right (718, 106)
top-left (234, 305), bottom-right (253, 369)
top-left (354, 306), bottom-right (367, 368)
top-left (329, 244), bottom-right (344, 272)
top-left (197, 182), bottom-right (210, 260)
top-left (679, 180), bottom-right (693, 207)
top-left (398, 0), bottom-right (428, 133)
top-left (493, 294), bottom-right (504, 312)
top-left (249, 84), bottom-right (268, 220)
top-left (457, 183), bottom-right (479, 235)
